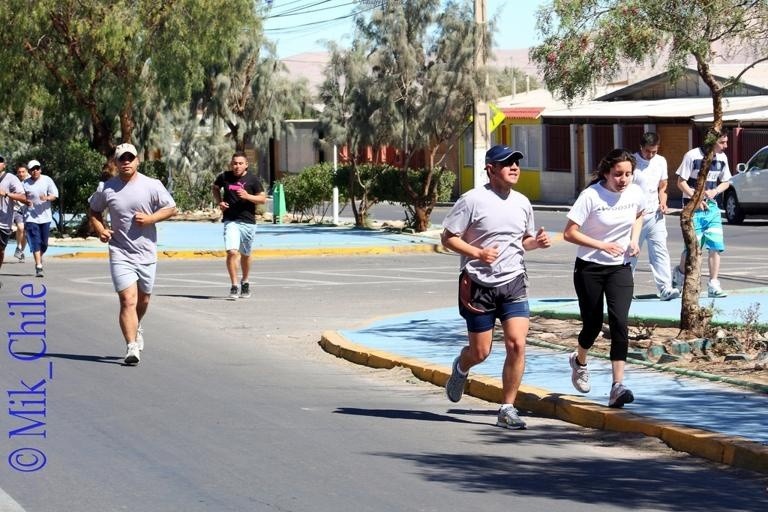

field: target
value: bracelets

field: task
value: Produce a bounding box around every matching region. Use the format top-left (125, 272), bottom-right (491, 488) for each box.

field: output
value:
top-left (715, 187), bottom-right (721, 197)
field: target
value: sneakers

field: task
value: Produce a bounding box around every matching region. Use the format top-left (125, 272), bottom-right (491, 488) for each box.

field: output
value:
top-left (447, 357), bottom-right (468, 402)
top-left (19, 253), bottom-right (24, 263)
top-left (608, 385), bottom-right (634, 406)
top-left (570, 353), bottom-right (591, 393)
top-left (14, 247), bottom-right (22, 259)
top-left (124, 344), bottom-right (140, 366)
top-left (659, 287), bottom-right (680, 301)
top-left (708, 279), bottom-right (727, 298)
top-left (496, 404), bottom-right (525, 430)
top-left (229, 287), bottom-right (239, 298)
top-left (35, 265), bottom-right (43, 277)
top-left (672, 269), bottom-right (686, 295)
top-left (240, 281), bottom-right (250, 297)
top-left (136, 330), bottom-right (144, 350)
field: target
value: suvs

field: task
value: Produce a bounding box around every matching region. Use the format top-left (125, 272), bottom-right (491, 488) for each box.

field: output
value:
top-left (722, 145), bottom-right (768, 224)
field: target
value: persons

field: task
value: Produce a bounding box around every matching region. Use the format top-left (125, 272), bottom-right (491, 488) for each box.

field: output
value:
top-left (628, 133), bottom-right (680, 302)
top-left (441, 145), bottom-right (551, 432)
top-left (89, 143), bottom-right (177, 366)
top-left (672, 126), bottom-right (731, 297)
top-left (212, 151), bottom-right (266, 303)
top-left (12, 164), bottom-right (30, 262)
top-left (567, 149), bottom-right (645, 409)
top-left (0, 153), bottom-right (26, 273)
top-left (22, 159), bottom-right (59, 278)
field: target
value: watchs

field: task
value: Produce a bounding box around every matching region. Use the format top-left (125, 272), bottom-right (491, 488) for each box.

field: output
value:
top-left (4, 191), bottom-right (8, 198)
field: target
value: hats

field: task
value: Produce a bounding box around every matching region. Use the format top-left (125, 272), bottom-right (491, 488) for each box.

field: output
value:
top-left (27, 160), bottom-right (40, 169)
top-left (0, 154), bottom-right (5, 160)
top-left (485, 144), bottom-right (524, 164)
top-left (114, 143), bottom-right (137, 159)
